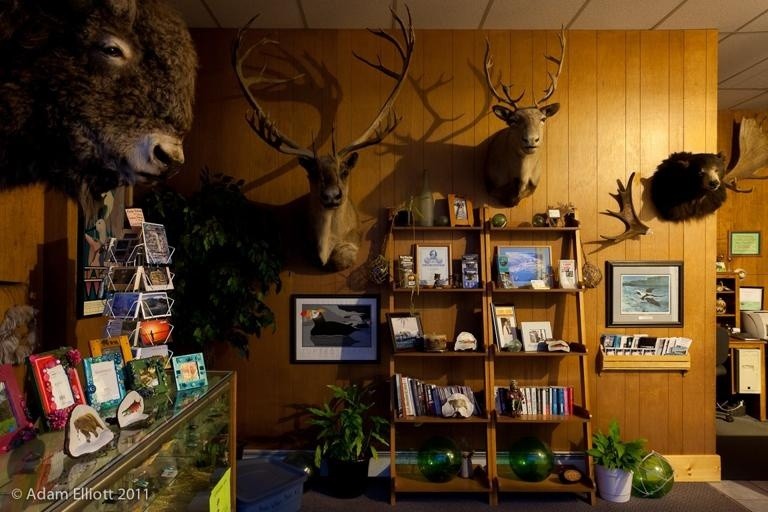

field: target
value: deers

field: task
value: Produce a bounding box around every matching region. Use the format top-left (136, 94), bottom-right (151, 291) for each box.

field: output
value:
top-left (483, 24), bottom-right (565, 208)
top-left (232, 3), bottom-right (416, 269)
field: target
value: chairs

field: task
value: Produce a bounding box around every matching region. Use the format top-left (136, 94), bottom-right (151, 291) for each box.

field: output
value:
top-left (717, 327), bottom-right (734, 421)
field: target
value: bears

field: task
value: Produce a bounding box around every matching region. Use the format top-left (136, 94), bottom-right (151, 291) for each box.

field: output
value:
top-left (650, 151), bottom-right (728, 223)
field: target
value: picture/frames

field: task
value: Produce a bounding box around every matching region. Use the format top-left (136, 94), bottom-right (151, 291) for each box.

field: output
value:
top-left (82, 352), bottom-right (127, 412)
top-left (386, 312), bottom-right (425, 352)
top-left (89, 336), bottom-right (133, 366)
top-left (520, 321), bottom-right (553, 351)
top-left (290, 294), bottom-right (381, 365)
top-left (416, 244), bottom-right (453, 288)
top-left (129, 356), bottom-right (167, 398)
top-left (107, 265), bottom-right (143, 290)
top-left (1, 366), bottom-right (32, 453)
top-left (497, 246), bottom-right (552, 288)
top-left (491, 303), bottom-right (519, 352)
top-left (29, 348), bottom-right (86, 429)
top-left (172, 352), bottom-right (209, 391)
top-left (728, 230), bottom-right (763, 257)
top-left (605, 261), bottom-right (683, 327)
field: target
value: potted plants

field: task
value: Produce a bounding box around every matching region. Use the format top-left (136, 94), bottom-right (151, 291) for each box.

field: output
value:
top-left (305, 382), bottom-right (390, 498)
top-left (586, 418), bottom-right (650, 505)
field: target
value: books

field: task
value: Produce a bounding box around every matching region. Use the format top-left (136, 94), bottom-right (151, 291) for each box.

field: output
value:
top-left (394, 371), bottom-right (576, 418)
top-left (602, 334), bottom-right (692, 355)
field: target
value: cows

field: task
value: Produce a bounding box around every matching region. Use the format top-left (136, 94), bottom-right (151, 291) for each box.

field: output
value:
top-left (0, 0), bottom-right (196, 194)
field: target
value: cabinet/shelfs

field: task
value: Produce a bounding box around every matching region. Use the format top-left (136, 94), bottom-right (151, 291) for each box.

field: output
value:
top-left (716, 274), bottom-right (739, 331)
top-left (387, 204), bottom-right (495, 508)
top-left (1, 371), bottom-right (237, 511)
top-left (485, 204), bottom-right (598, 508)
